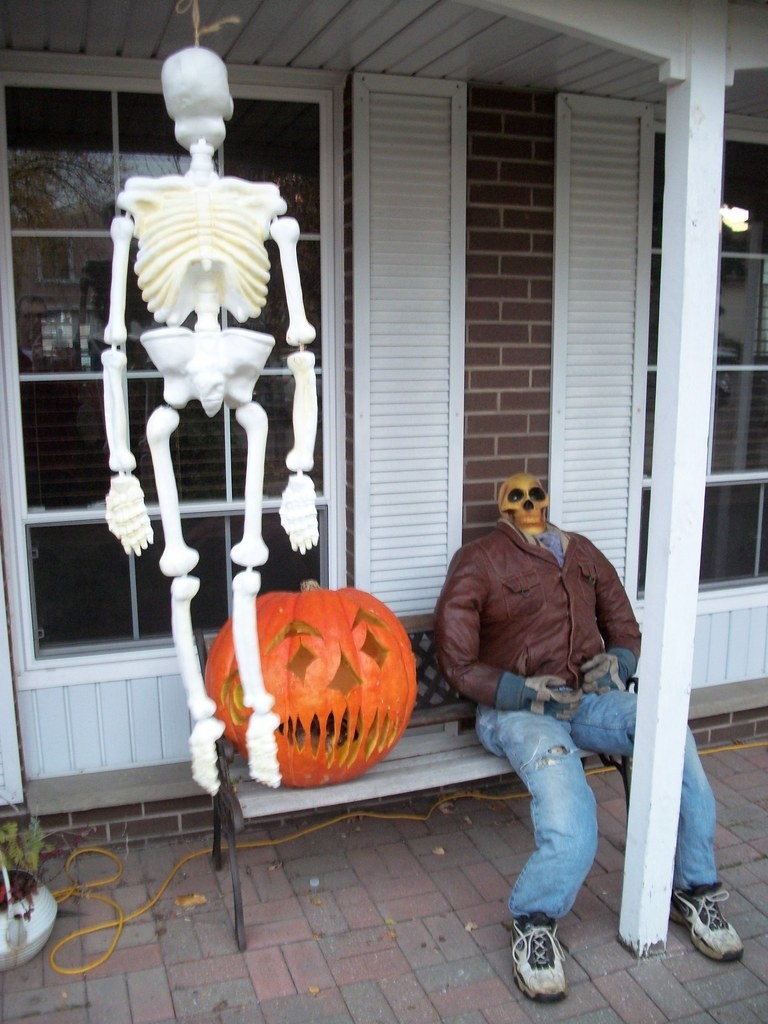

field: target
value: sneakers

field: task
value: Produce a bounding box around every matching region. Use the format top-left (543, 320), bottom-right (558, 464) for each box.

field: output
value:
top-left (509, 913), bottom-right (567, 1000)
top-left (669, 885), bottom-right (744, 964)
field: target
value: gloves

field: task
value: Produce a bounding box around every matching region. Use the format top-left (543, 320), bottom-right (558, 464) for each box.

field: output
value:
top-left (493, 672), bottom-right (582, 721)
top-left (580, 647), bottom-right (636, 693)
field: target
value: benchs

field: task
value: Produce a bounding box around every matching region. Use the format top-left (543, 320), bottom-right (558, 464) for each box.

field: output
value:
top-left (202, 613), bottom-right (639, 952)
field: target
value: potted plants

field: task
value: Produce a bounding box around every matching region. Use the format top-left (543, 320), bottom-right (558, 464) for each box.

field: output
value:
top-left (0, 817), bottom-right (56, 972)
